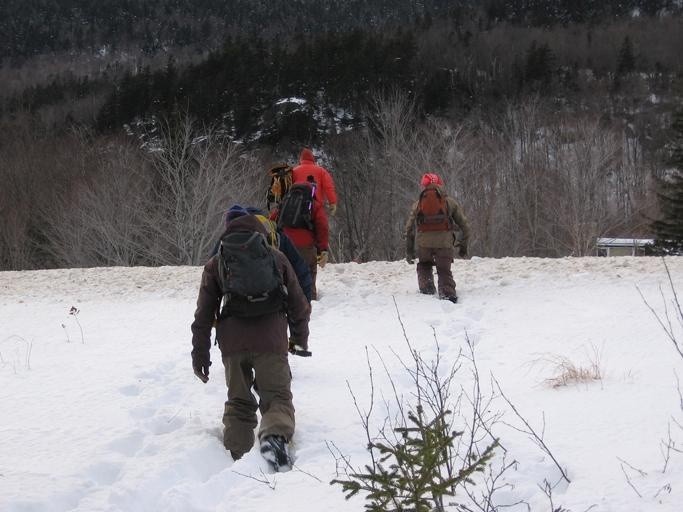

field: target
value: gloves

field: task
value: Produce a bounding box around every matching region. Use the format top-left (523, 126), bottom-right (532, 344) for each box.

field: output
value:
top-left (406, 252), bottom-right (415, 264)
top-left (454, 243), bottom-right (466, 257)
top-left (288, 339), bottom-right (310, 356)
top-left (319, 251), bottom-right (329, 267)
top-left (193, 362), bottom-right (209, 382)
top-left (329, 204), bottom-right (337, 216)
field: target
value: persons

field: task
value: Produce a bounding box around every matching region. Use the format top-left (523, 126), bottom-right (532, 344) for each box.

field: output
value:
top-left (244, 207), bottom-right (313, 318)
top-left (285, 147), bottom-right (338, 220)
top-left (188, 204), bottom-right (312, 475)
top-left (265, 182), bottom-right (331, 303)
top-left (403, 172), bottom-right (471, 306)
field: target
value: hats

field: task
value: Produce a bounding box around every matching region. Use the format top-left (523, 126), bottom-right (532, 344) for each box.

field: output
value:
top-left (419, 173), bottom-right (443, 190)
top-left (300, 147), bottom-right (314, 160)
top-left (225, 205), bottom-right (249, 227)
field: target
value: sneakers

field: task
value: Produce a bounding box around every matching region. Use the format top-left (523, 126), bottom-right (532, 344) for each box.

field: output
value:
top-left (260, 433), bottom-right (292, 472)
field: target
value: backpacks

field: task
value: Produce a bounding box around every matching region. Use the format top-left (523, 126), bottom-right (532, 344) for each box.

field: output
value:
top-left (414, 184), bottom-right (453, 232)
top-left (278, 181), bottom-right (315, 231)
top-left (218, 232), bottom-right (288, 319)
top-left (265, 162), bottom-right (293, 202)
